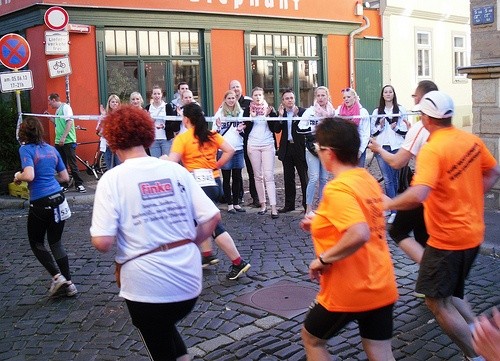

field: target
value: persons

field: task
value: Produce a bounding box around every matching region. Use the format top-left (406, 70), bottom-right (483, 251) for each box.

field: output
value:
top-left (228, 80), bottom-right (260, 208)
top-left (241, 87), bottom-right (282, 219)
top-left (298, 116), bottom-right (399, 361)
top-left (371, 83), bottom-right (409, 225)
top-left (44, 93), bottom-right (87, 194)
top-left (211, 90), bottom-right (247, 213)
top-left (334, 87), bottom-right (371, 171)
top-left (377, 90), bottom-right (500, 361)
top-left (96, 81), bottom-right (201, 171)
top-left (366, 80), bottom-right (439, 297)
top-left (87, 106), bottom-right (221, 361)
top-left (299, 85), bottom-right (336, 217)
top-left (471, 307), bottom-right (500, 361)
top-left (273, 89), bottom-right (310, 215)
top-left (13, 117), bottom-right (78, 298)
top-left (163, 102), bottom-right (252, 281)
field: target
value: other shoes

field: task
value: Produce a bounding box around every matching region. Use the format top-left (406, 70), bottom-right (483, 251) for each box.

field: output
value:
top-left (226, 260), bottom-right (252, 280)
top-left (62, 283), bottom-right (79, 296)
top-left (60, 187), bottom-right (69, 193)
top-left (74, 185), bottom-right (87, 193)
top-left (252, 197), bottom-right (261, 208)
top-left (227, 208), bottom-right (236, 214)
top-left (202, 251), bottom-right (220, 269)
top-left (271, 209), bottom-right (280, 219)
top-left (278, 206), bottom-right (295, 214)
top-left (48, 275), bottom-right (67, 297)
top-left (234, 206), bottom-right (247, 212)
top-left (257, 207), bottom-right (268, 215)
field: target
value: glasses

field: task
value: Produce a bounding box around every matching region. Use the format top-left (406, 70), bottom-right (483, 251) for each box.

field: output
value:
top-left (341, 89), bottom-right (351, 92)
top-left (313, 142), bottom-right (340, 152)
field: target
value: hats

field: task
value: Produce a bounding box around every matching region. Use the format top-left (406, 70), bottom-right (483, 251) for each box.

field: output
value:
top-left (409, 90), bottom-right (454, 119)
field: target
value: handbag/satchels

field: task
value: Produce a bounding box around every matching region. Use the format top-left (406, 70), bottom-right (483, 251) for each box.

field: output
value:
top-left (305, 132), bottom-right (320, 158)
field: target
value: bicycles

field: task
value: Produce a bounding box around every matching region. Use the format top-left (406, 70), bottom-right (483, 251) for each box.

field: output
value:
top-left (59, 124), bottom-right (107, 189)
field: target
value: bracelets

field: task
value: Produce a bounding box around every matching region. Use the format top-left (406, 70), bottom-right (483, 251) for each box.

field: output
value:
top-left (317, 254), bottom-right (331, 266)
